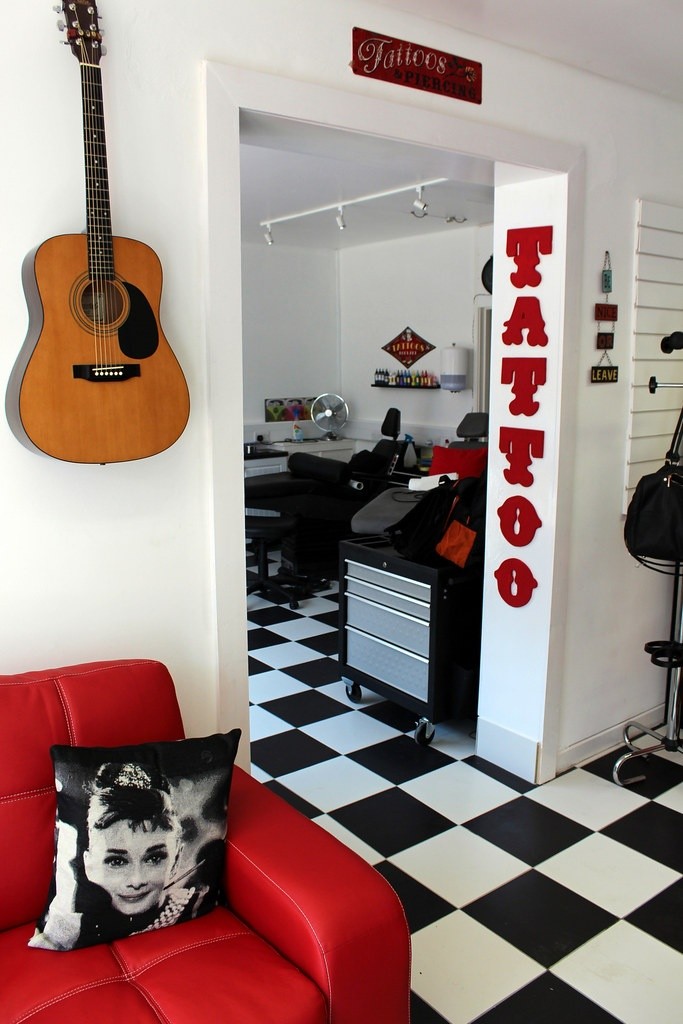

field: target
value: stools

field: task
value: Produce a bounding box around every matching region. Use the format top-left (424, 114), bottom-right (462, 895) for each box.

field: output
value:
top-left (245, 516), bottom-right (330, 610)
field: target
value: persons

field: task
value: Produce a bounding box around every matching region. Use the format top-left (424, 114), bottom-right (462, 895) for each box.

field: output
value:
top-left (83, 764), bottom-right (215, 938)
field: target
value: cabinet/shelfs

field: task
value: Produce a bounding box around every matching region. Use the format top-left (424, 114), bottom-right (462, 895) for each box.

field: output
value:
top-left (337, 534), bottom-right (484, 749)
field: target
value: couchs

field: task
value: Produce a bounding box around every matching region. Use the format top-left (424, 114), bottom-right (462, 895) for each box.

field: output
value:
top-left (0, 658), bottom-right (411, 1024)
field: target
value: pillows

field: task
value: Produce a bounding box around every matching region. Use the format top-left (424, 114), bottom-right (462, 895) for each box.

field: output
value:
top-left (29, 728), bottom-right (244, 951)
top-left (429, 445), bottom-right (488, 478)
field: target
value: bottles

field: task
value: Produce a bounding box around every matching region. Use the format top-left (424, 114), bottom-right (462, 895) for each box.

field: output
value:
top-left (375, 368), bottom-right (434, 387)
top-left (292, 409), bottom-right (304, 442)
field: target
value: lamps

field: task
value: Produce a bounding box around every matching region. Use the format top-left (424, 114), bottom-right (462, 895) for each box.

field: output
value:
top-left (413, 189), bottom-right (427, 211)
top-left (335, 208), bottom-right (344, 230)
top-left (263, 225), bottom-right (273, 245)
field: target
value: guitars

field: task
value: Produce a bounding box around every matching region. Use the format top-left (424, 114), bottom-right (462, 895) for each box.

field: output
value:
top-left (17, 0), bottom-right (193, 467)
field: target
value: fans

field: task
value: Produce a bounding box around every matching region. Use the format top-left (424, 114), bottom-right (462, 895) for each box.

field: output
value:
top-left (310, 393), bottom-right (349, 441)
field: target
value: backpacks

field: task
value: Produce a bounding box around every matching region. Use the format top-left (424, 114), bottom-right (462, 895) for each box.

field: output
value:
top-left (386, 475), bottom-right (486, 573)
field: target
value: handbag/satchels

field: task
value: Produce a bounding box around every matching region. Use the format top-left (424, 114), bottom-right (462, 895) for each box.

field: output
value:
top-left (622, 463), bottom-right (683, 563)
